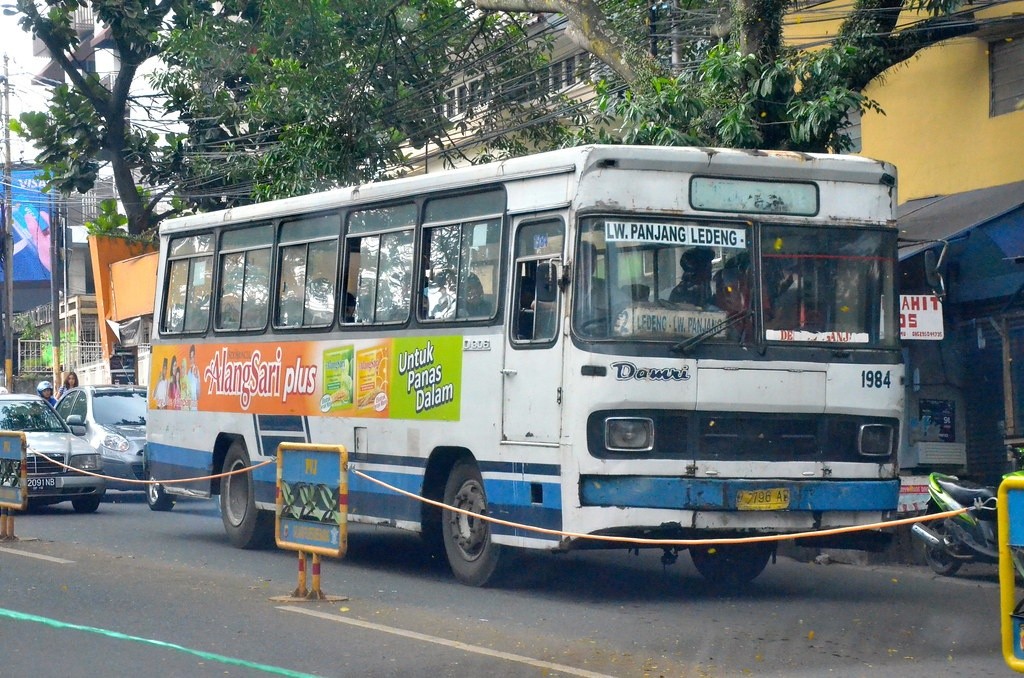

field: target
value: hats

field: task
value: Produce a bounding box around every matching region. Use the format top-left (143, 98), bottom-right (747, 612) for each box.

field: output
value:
top-left (681, 245), bottom-right (715, 281)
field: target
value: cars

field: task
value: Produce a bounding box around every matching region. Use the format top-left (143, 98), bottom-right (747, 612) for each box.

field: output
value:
top-left (0, 385), bottom-right (107, 518)
top-left (51, 383), bottom-right (174, 506)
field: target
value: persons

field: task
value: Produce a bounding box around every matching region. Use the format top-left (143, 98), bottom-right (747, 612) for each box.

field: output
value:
top-left (35, 381), bottom-right (58, 409)
top-left (668, 248), bottom-right (718, 306)
top-left (559, 241), bottom-right (634, 325)
top-left (55, 372), bottom-right (79, 401)
top-left (174, 270), bottom-right (555, 332)
top-left (152, 345), bottom-right (202, 412)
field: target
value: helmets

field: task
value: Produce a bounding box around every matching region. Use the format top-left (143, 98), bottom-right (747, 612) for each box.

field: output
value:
top-left (37, 381), bottom-right (54, 398)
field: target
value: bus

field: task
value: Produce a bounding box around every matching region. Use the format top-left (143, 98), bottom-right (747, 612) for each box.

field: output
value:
top-left (141, 146), bottom-right (952, 589)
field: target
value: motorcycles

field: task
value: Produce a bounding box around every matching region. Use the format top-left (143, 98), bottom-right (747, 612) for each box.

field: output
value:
top-left (912, 472), bottom-right (1021, 579)
